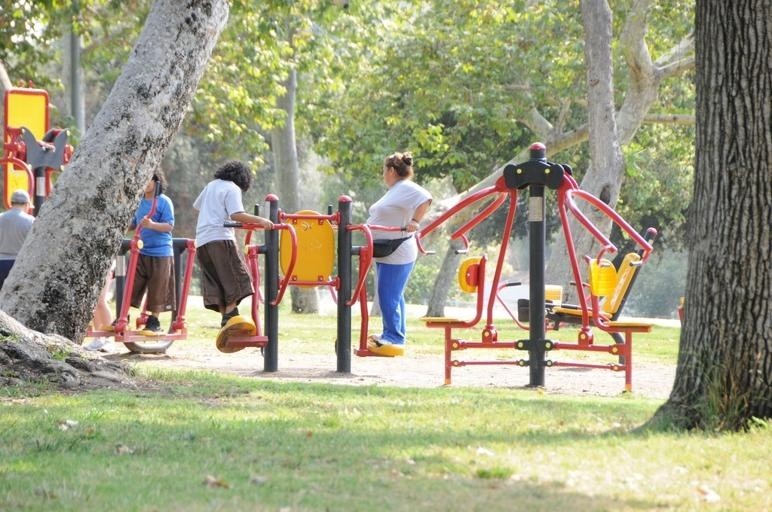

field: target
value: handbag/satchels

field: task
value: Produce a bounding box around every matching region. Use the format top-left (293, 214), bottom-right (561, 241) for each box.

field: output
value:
top-left (372, 235), bottom-right (413, 258)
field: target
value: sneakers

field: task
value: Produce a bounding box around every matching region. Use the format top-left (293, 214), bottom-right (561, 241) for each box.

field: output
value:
top-left (367, 334), bottom-right (391, 347)
top-left (221, 307), bottom-right (238, 326)
top-left (142, 315), bottom-right (161, 334)
top-left (82, 338), bottom-right (112, 352)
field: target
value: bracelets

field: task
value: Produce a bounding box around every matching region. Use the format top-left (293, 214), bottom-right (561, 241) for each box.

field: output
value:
top-left (413, 218), bottom-right (421, 224)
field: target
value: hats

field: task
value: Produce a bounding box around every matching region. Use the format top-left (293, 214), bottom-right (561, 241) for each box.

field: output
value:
top-left (10, 189), bottom-right (34, 208)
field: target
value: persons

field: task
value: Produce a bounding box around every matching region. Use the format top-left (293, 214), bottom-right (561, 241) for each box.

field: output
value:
top-left (192, 160), bottom-right (275, 328)
top-left (360, 152), bottom-right (435, 351)
top-left (112, 166), bottom-right (177, 334)
top-left (0, 189), bottom-right (36, 289)
top-left (84, 257), bottom-right (117, 352)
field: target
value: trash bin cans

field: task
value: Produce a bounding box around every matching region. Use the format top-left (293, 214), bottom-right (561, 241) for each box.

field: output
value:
top-left (518, 299), bottom-right (529, 322)
